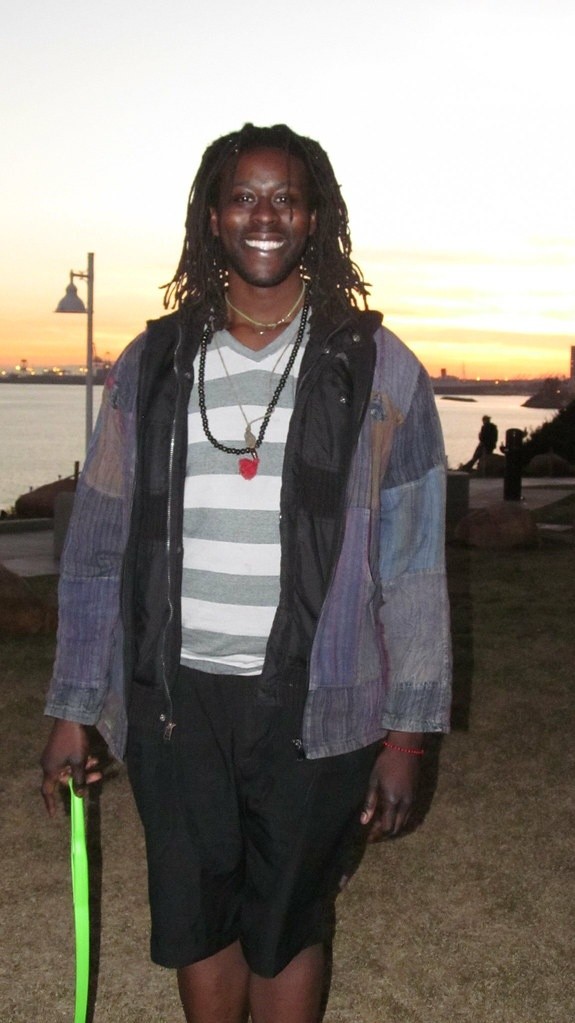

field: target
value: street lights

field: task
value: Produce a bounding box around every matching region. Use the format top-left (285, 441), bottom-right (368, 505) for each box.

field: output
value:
top-left (54, 251), bottom-right (94, 451)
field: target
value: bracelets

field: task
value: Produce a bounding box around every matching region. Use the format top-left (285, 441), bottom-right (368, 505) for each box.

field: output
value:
top-left (383, 740), bottom-right (424, 755)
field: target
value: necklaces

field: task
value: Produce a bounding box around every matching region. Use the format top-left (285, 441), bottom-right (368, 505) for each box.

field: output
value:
top-left (198, 276), bottom-right (310, 480)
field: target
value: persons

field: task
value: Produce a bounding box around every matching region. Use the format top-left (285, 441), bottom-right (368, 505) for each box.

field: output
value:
top-left (42, 125), bottom-right (453, 1022)
top-left (463, 416), bottom-right (498, 470)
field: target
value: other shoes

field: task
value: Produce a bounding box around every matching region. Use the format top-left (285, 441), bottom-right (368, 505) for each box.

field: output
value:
top-left (461, 463), bottom-right (470, 472)
